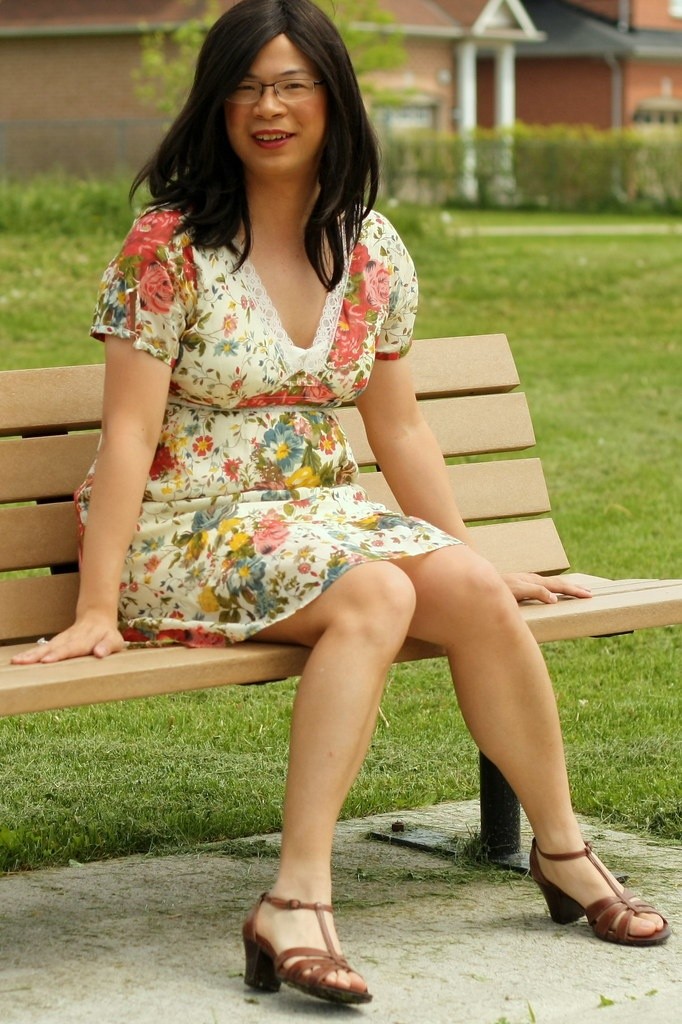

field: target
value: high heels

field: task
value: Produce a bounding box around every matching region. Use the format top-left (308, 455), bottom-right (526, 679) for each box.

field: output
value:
top-left (528, 835), bottom-right (671, 946)
top-left (242, 893), bottom-right (372, 1004)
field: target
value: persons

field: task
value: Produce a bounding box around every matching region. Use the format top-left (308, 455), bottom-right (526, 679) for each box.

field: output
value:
top-left (8, 0), bottom-right (672, 1003)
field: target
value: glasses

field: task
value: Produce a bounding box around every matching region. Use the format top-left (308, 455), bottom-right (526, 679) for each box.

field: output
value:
top-left (223, 78), bottom-right (323, 104)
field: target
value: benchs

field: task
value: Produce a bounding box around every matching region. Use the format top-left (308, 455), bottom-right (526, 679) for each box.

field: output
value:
top-left (0, 333), bottom-right (682, 887)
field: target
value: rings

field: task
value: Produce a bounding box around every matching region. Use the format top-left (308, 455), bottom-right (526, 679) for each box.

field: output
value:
top-left (34, 637), bottom-right (47, 646)
